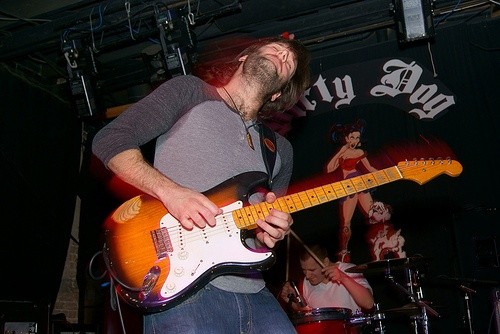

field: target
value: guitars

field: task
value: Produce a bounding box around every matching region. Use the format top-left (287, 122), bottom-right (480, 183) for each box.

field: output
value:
top-left (102, 158), bottom-right (465, 312)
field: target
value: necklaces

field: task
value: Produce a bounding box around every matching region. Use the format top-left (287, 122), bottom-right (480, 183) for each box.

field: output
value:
top-left (221, 85), bottom-right (262, 150)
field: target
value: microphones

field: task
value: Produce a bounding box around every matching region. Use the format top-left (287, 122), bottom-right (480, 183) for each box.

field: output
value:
top-left (291, 280), bottom-right (307, 308)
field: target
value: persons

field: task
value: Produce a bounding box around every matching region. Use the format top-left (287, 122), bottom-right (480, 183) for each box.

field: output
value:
top-left (282, 243), bottom-right (375, 316)
top-left (91, 36), bottom-right (310, 334)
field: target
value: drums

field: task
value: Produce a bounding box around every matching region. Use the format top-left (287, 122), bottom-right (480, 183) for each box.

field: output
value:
top-left (290, 307), bottom-right (369, 334)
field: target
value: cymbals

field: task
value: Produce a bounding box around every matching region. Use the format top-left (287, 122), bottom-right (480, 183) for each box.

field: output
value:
top-left (386, 304), bottom-right (440, 312)
top-left (344, 255), bottom-right (425, 274)
